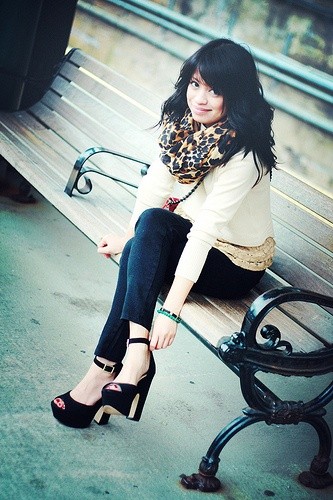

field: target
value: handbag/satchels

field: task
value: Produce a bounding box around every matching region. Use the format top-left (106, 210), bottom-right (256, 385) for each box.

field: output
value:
top-left (162, 197), bottom-right (181, 213)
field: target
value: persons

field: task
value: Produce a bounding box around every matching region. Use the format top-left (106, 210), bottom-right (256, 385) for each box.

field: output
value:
top-left (51, 38), bottom-right (282, 428)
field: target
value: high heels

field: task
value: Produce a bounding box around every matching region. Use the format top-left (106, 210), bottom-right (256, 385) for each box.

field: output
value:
top-left (101, 337), bottom-right (157, 423)
top-left (51, 356), bottom-right (123, 429)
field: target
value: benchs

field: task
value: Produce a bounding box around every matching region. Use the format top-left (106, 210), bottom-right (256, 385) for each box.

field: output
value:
top-left (0, 48), bottom-right (333, 493)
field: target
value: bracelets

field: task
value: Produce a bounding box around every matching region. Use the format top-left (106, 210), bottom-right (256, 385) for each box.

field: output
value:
top-left (157, 308), bottom-right (181, 324)
